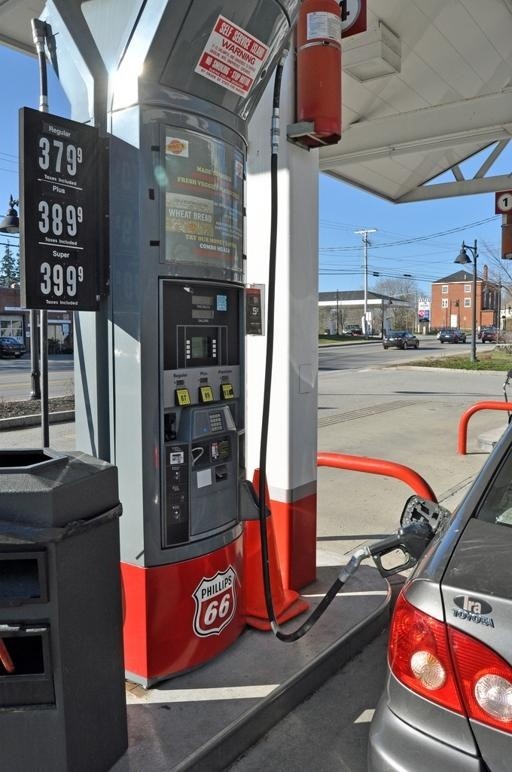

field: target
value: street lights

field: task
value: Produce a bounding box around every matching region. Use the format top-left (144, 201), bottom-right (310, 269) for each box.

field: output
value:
top-left (455, 239), bottom-right (479, 363)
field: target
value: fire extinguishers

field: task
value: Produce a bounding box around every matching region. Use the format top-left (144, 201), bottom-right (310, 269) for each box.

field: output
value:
top-left (296, 0), bottom-right (342, 146)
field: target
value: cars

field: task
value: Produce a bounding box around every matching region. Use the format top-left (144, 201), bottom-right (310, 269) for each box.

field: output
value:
top-left (369, 368), bottom-right (512, 772)
top-left (342, 324), bottom-right (361, 336)
top-left (481, 328), bottom-right (510, 344)
top-left (438, 328), bottom-right (467, 344)
top-left (382, 330), bottom-right (419, 350)
top-left (478, 326), bottom-right (496, 339)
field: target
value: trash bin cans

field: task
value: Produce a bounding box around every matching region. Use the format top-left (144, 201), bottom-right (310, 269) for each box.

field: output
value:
top-left (1, 449), bottom-right (125, 770)
